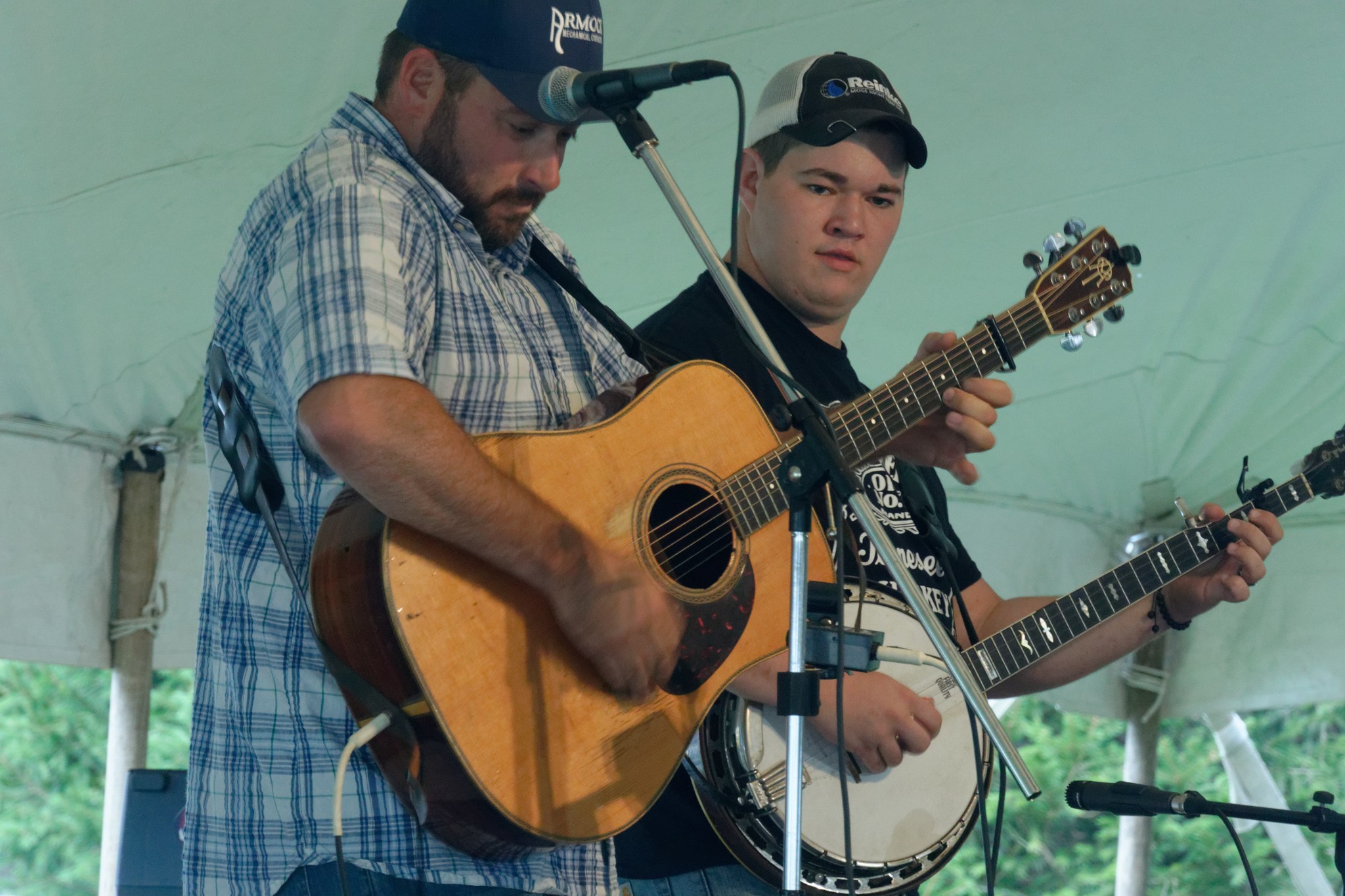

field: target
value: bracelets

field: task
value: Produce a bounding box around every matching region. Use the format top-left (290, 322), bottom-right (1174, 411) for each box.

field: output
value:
top-left (1147, 588), bottom-right (1192, 634)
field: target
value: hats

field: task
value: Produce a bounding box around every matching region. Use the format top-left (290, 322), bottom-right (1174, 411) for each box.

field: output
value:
top-left (742, 52), bottom-right (929, 169)
top-left (395, 0), bottom-right (617, 124)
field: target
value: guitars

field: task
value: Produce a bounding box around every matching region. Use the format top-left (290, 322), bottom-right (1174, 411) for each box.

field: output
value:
top-left (305, 214), bottom-right (1146, 865)
top-left (693, 420), bottom-right (1345, 896)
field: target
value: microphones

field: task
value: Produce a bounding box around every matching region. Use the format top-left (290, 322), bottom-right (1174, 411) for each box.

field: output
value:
top-left (537, 58), bottom-right (731, 121)
top-left (1064, 780), bottom-right (1218, 817)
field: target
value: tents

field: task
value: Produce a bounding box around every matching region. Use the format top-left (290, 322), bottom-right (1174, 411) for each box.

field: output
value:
top-left (1, 0), bottom-right (1343, 896)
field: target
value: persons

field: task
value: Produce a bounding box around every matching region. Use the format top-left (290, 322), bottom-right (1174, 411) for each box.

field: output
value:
top-left (634, 48), bottom-right (1282, 896)
top-left (171, 1), bottom-right (1014, 896)
